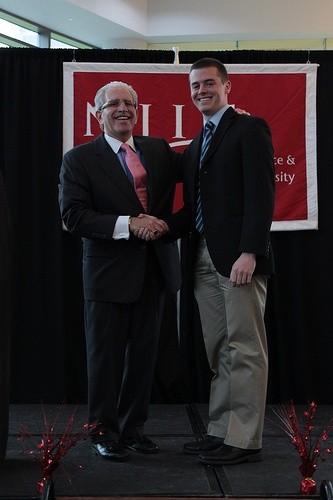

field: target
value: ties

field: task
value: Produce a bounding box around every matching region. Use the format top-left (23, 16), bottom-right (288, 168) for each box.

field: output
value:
top-left (195, 120), bottom-right (216, 233)
top-left (120, 143), bottom-right (149, 213)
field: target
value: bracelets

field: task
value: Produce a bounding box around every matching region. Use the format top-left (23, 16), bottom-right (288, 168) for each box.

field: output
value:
top-left (128, 217), bottom-right (132, 232)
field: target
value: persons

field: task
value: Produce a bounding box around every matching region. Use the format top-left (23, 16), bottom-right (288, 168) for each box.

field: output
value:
top-left (134, 58), bottom-right (276, 466)
top-left (59, 81), bottom-right (250, 462)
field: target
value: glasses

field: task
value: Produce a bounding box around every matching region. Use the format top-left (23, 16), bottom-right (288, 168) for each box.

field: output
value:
top-left (100, 98), bottom-right (136, 111)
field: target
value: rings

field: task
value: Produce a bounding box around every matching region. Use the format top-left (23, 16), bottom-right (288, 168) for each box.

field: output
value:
top-left (154, 230), bottom-right (158, 235)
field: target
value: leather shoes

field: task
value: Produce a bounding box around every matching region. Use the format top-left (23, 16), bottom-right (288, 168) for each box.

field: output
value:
top-left (196, 443), bottom-right (265, 465)
top-left (87, 438), bottom-right (131, 462)
top-left (182, 435), bottom-right (225, 454)
top-left (121, 428), bottom-right (160, 454)
